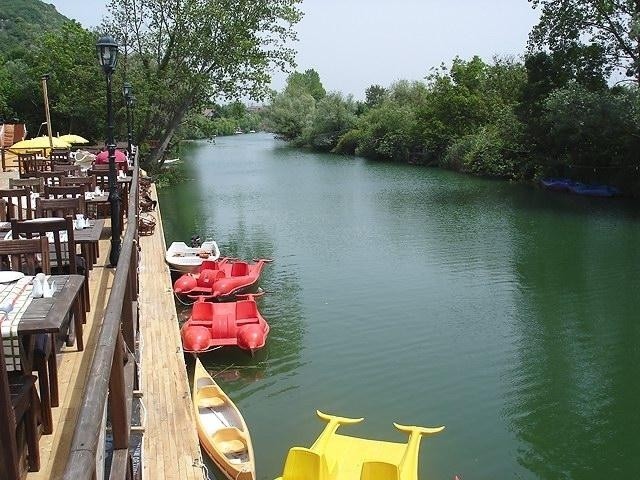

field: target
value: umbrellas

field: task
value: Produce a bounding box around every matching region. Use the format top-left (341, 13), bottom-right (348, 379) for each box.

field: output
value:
top-left (9, 132), bottom-right (90, 155)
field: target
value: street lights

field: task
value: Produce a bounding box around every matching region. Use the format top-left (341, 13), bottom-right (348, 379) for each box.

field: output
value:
top-left (120, 76), bottom-right (137, 164)
top-left (95, 29), bottom-right (125, 272)
top-left (40, 72), bottom-right (53, 150)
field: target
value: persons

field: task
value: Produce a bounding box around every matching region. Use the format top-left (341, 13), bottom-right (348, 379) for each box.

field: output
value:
top-left (94, 140), bottom-right (128, 171)
top-left (74, 147), bottom-right (95, 170)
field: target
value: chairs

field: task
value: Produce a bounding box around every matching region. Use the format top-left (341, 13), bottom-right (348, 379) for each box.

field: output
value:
top-left (1, 147), bottom-right (135, 480)
top-left (174, 243), bottom-right (259, 328)
top-left (139, 178), bottom-right (157, 236)
top-left (282, 446), bottom-right (400, 480)
top-left (197, 385), bottom-right (248, 458)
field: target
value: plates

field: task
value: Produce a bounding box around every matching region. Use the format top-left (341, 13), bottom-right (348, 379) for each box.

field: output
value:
top-left (0, 270), bottom-right (24, 283)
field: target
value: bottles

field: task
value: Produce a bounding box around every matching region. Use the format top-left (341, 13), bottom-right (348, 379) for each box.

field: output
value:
top-left (95, 185), bottom-right (104, 196)
top-left (119, 170), bottom-right (127, 179)
top-left (32, 273), bottom-right (55, 299)
top-left (75, 214), bottom-right (90, 230)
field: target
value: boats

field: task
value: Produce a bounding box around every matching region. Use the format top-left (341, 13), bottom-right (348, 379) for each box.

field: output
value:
top-left (540, 175), bottom-right (624, 200)
top-left (270, 409), bottom-right (446, 479)
top-left (164, 233), bottom-right (223, 276)
top-left (178, 293), bottom-right (273, 364)
top-left (173, 258), bottom-right (276, 303)
top-left (191, 356), bottom-right (257, 480)
